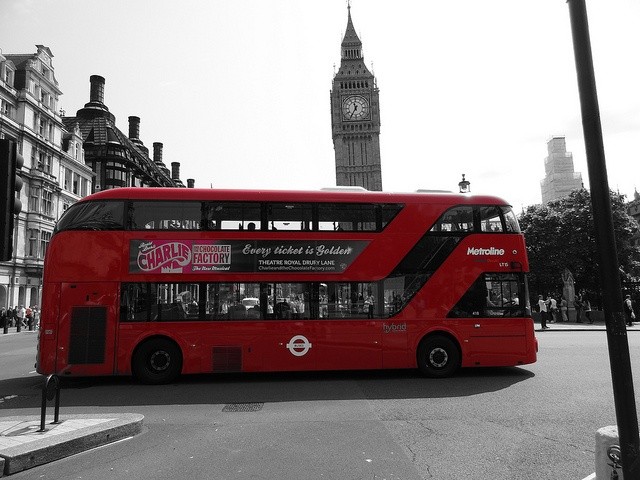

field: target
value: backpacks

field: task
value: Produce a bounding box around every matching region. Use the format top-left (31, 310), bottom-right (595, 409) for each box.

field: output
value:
top-left (624, 299), bottom-right (631, 312)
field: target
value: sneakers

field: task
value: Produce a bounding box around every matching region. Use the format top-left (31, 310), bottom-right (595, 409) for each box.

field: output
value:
top-left (24, 326), bottom-right (27, 329)
top-left (543, 326), bottom-right (546, 329)
top-left (546, 326), bottom-right (549, 328)
top-left (590, 319), bottom-right (593, 323)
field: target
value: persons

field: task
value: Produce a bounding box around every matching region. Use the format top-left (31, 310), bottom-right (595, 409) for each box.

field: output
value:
top-left (583, 298), bottom-right (594, 324)
top-left (548, 296), bottom-right (558, 323)
top-left (248, 223), bottom-right (255, 231)
top-left (545, 295), bottom-right (552, 323)
top-left (559, 296), bottom-right (569, 322)
top-left (538, 295), bottom-right (551, 330)
top-left (291, 296), bottom-right (305, 319)
top-left (358, 290), bottom-right (375, 320)
top-left (0, 304), bottom-right (41, 334)
top-left (486, 288), bottom-right (504, 317)
top-left (574, 293), bottom-right (584, 323)
top-left (512, 293), bottom-right (520, 305)
top-left (624, 294), bottom-right (634, 326)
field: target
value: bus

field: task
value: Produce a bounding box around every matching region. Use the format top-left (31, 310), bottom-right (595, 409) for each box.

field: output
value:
top-left (34, 185), bottom-right (540, 386)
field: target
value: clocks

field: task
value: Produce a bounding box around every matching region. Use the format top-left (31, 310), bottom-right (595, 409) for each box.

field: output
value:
top-left (341, 93), bottom-right (370, 121)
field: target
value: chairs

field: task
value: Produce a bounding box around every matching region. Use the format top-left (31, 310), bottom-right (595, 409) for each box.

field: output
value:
top-left (248, 308), bottom-right (260, 318)
top-left (273, 303), bottom-right (289, 318)
top-left (228, 305), bottom-right (246, 319)
top-left (248, 222), bottom-right (255, 228)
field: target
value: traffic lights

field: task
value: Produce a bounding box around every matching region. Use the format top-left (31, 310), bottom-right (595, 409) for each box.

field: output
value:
top-left (0, 138), bottom-right (25, 263)
